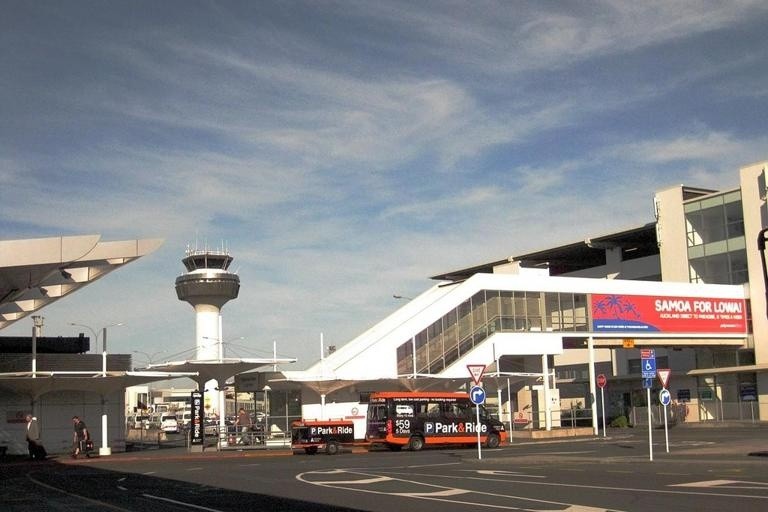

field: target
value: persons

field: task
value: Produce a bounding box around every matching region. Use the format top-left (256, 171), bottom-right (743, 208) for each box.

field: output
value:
top-left (72, 415), bottom-right (91, 458)
top-left (235, 407), bottom-right (252, 445)
top-left (24, 413), bottom-right (40, 460)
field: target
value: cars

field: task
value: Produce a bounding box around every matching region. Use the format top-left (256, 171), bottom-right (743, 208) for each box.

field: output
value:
top-left (204, 411), bottom-right (269, 436)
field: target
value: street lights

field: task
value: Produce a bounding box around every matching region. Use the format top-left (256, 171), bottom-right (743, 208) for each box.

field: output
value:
top-left (70, 320), bottom-right (126, 371)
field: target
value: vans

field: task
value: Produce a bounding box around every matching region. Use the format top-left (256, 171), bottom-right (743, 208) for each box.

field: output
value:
top-left (126, 405), bottom-right (192, 434)
top-left (367, 390), bottom-right (509, 456)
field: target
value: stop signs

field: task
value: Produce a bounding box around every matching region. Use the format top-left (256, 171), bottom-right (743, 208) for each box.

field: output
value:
top-left (597, 375), bottom-right (607, 388)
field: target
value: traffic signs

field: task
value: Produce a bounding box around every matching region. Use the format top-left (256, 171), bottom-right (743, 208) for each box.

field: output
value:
top-left (640, 348), bottom-right (656, 378)
